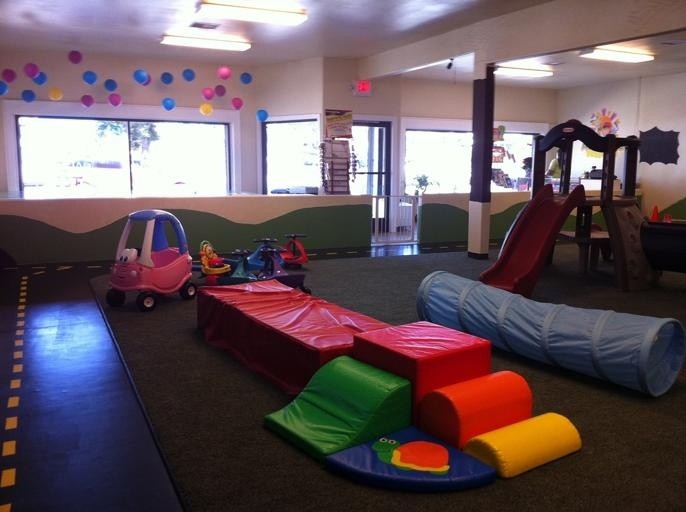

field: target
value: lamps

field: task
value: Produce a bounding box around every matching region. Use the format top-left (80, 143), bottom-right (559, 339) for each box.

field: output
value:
top-left (577, 47), bottom-right (655, 64)
top-left (194, 0), bottom-right (309, 29)
top-left (490, 66), bottom-right (555, 78)
top-left (160, 34), bottom-right (253, 53)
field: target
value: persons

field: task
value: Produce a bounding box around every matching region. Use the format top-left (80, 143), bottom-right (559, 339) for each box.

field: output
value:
top-left (545, 148), bottom-right (563, 178)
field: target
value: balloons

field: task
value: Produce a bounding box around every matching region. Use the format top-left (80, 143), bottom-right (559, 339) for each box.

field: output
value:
top-left (68, 50), bottom-right (83, 64)
top-left (0, 62), bottom-right (231, 117)
top-left (240, 72), bottom-right (252, 85)
top-left (255, 109), bottom-right (268, 123)
top-left (232, 97), bottom-right (244, 111)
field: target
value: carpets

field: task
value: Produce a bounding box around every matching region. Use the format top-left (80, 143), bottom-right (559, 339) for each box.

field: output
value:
top-left (87, 242), bottom-right (685, 511)
